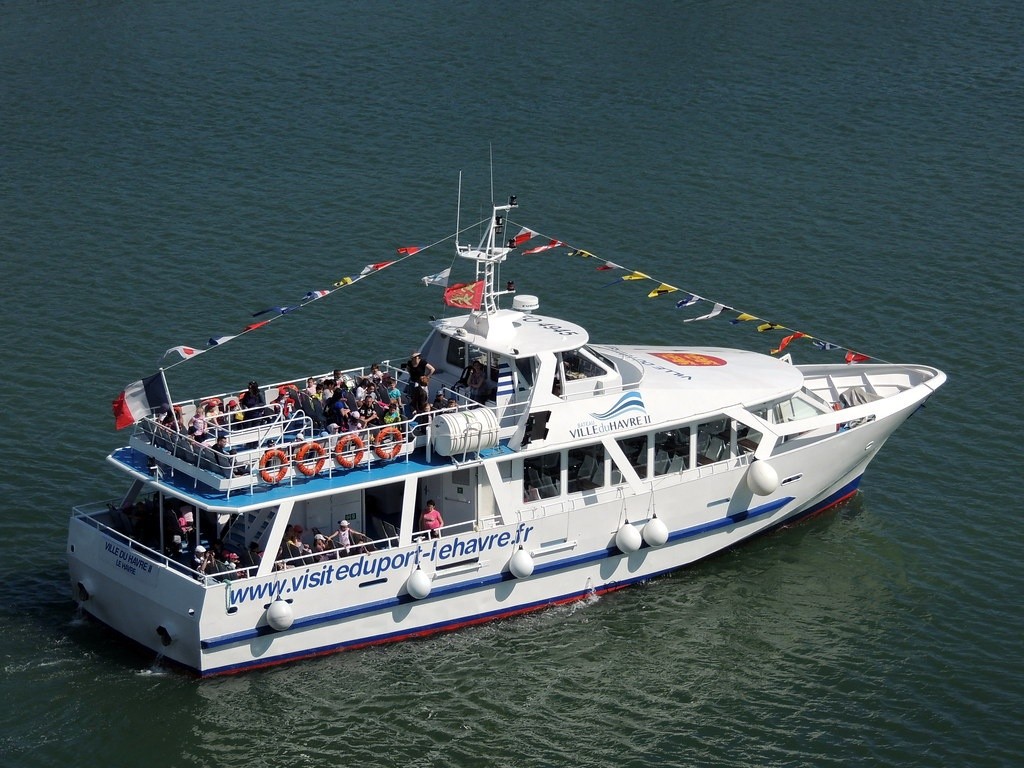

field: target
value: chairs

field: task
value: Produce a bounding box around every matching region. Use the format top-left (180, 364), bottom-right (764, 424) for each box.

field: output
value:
top-left (106, 494), bottom-right (401, 582)
top-left (140, 420), bottom-right (249, 493)
top-left (524, 417), bottom-right (761, 502)
top-left (223, 396), bottom-right (239, 424)
top-left (291, 388), bottom-right (417, 443)
top-left (181, 404), bottom-right (198, 431)
top-left (264, 388), bottom-right (280, 411)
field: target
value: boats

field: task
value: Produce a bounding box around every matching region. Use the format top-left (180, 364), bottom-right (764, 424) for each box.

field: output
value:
top-left (64, 140), bottom-right (947, 671)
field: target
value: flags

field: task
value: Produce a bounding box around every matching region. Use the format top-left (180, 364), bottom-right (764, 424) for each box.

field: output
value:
top-left (162, 246), bottom-right (428, 361)
top-left (443, 280), bottom-right (485, 310)
top-left (506, 226), bottom-right (871, 367)
top-left (422, 267), bottom-right (451, 288)
top-left (112, 372), bottom-right (171, 432)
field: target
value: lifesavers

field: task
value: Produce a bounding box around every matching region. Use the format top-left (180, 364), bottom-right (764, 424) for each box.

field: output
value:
top-left (335, 434), bottom-right (364, 468)
top-left (375, 427), bottom-right (403, 459)
top-left (296, 442), bottom-right (325, 476)
top-left (259, 450), bottom-right (289, 483)
top-left (198, 398), bottom-right (223, 423)
top-left (277, 384), bottom-right (299, 394)
top-left (157, 406), bottom-right (183, 430)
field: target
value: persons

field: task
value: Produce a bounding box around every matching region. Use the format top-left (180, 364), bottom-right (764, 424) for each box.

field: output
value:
top-left (326, 520), bottom-right (365, 553)
top-left (407, 352), bottom-right (435, 403)
top-left (419, 499), bottom-right (444, 540)
top-left (158, 390), bottom-right (253, 475)
top-left (270, 389), bottom-right (296, 434)
top-left (242, 381), bottom-right (266, 449)
top-left (130, 501), bottom-right (370, 583)
top-left (265, 432), bottom-right (304, 470)
top-left (305, 364), bottom-right (458, 457)
top-left (467, 360), bottom-right (489, 410)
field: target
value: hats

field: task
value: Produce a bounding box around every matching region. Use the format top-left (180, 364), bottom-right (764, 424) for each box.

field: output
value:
top-left (314, 534), bottom-right (326, 541)
top-left (230, 400), bottom-right (236, 407)
top-left (195, 546), bottom-right (206, 553)
top-left (330, 423), bottom-right (339, 429)
top-left (208, 403), bottom-right (216, 408)
top-left (296, 433), bottom-right (304, 440)
top-left (411, 352), bottom-right (421, 358)
top-left (267, 440), bottom-right (274, 445)
top-left (340, 520), bottom-right (350, 526)
top-left (279, 390), bottom-right (286, 395)
top-left (230, 553), bottom-right (239, 559)
top-left (353, 411), bottom-right (360, 419)
top-left (293, 525), bottom-right (303, 532)
